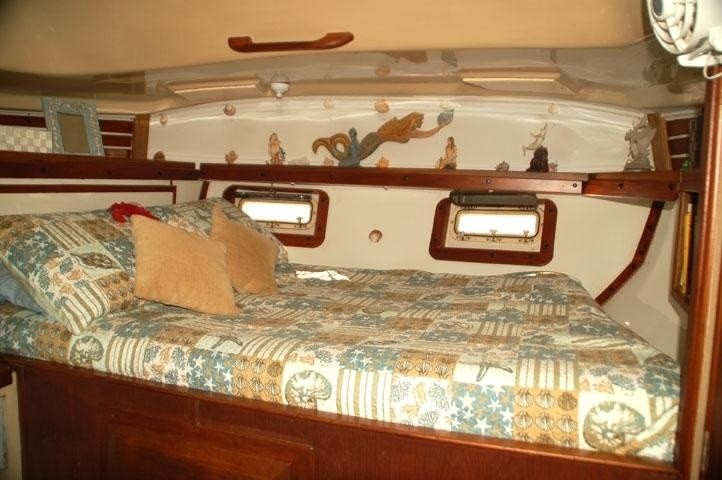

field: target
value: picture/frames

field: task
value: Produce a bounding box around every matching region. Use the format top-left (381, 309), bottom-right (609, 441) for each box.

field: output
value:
top-left (41, 95), bottom-right (106, 157)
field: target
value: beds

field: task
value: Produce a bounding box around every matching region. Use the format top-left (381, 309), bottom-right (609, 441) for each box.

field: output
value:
top-left (2, 262), bottom-right (681, 479)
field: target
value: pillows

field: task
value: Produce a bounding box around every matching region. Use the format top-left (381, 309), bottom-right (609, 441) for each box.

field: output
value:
top-left (1, 196), bottom-right (294, 323)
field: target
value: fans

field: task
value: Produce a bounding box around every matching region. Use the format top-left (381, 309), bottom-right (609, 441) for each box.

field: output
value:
top-left (645, 0), bottom-right (721, 79)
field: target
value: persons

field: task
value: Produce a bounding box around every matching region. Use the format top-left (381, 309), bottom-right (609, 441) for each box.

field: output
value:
top-left (438, 136), bottom-right (458, 169)
top-left (266, 132), bottom-right (282, 162)
